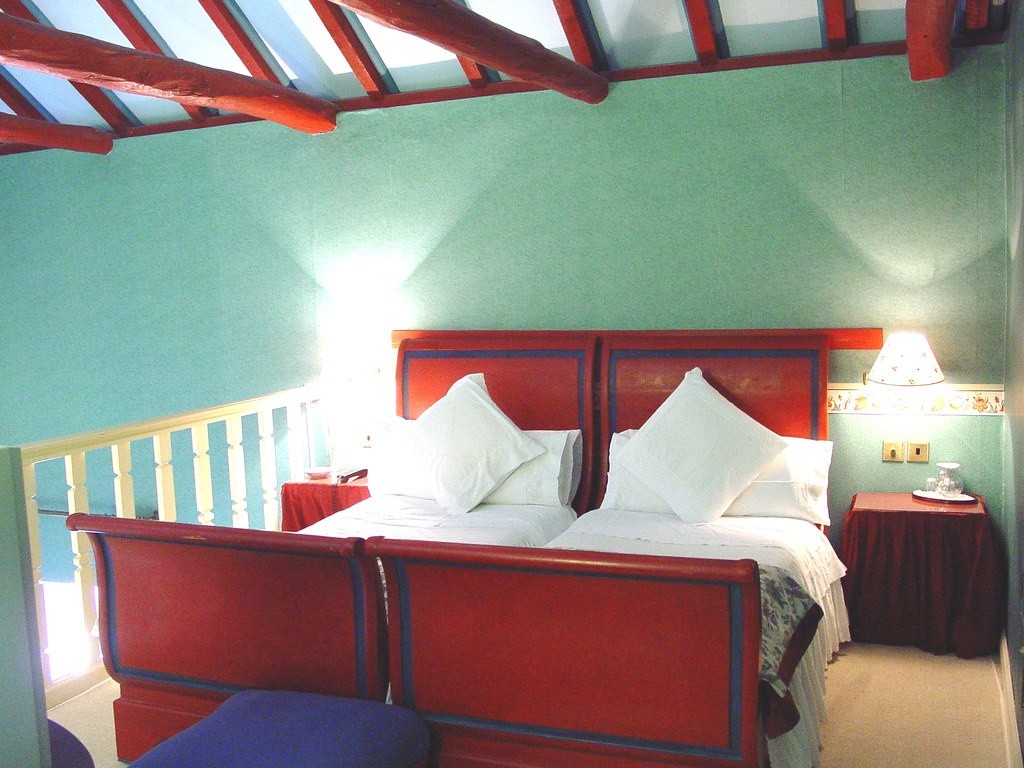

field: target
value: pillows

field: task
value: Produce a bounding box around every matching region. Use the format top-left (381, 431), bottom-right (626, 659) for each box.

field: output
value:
top-left (599, 427), bottom-right (835, 526)
top-left (394, 372), bottom-right (547, 514)
top-left (369, 423), bottom-right (583, 508)
top-left (609, 366), bottom-right (787, 523)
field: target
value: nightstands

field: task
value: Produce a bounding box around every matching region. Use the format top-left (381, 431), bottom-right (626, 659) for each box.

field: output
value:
top-left (847, 491), bottom-right (989, 654)
top-left (281, 470), bottom-right (371, 531)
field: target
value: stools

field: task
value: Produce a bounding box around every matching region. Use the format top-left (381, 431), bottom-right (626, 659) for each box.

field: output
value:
top-left (131, 689), bottom-right (431, 768)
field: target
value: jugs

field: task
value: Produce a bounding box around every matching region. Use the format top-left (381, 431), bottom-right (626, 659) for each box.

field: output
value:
top-left (935, 463), bottom-right (963, 498)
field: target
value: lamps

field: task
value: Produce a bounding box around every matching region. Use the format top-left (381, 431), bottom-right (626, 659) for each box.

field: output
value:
top-left (863, 331), bottom-right (945, 385)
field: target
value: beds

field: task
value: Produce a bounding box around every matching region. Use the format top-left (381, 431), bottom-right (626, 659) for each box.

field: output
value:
top-left (65, 330), bottom-right (830, 768)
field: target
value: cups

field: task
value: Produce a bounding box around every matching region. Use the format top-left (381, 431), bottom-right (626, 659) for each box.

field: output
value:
top-left (925, 478), bottom-right (937, 495)
